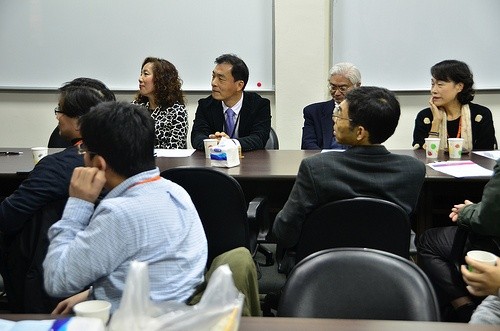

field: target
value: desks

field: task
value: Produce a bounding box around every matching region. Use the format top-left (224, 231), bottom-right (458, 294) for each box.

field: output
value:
top-left (0, 147), bottom-right (500, 241)
top-left (0, 313), bottom-right (500, 331)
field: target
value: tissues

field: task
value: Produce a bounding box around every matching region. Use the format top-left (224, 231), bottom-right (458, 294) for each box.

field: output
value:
top-left (208, 137), bottom-right (240, 168)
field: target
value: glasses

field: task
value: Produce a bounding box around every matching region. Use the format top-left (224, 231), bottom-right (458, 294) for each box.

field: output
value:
top-left (54, 107), bottom-right (63, 115)
top-left (77, 143), bottom-right (98, 156)
top-left (328, 82), bottom-right (350, 92)
top-left (332, 114), bottom-right (352, 123)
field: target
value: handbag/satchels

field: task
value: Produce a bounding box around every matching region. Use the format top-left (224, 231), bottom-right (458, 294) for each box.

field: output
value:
top-left (109, 260), bottom-right (245, 331)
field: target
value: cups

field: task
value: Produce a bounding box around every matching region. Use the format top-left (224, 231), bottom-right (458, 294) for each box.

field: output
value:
top-left (73, 300), bottom-right (112, 331)
top-left (466, 250), bottom-right (498, 273)
top-left (424, 138), bottom-right (441, 159)
top-left (447, 138), bottom-right (464, 159)
top-left (204, 139), bottom-right (219, 159)
top-left (31, 146), bottom-right (48, 166)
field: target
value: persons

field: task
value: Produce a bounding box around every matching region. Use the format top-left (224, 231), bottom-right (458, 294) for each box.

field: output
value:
top-left (301, 63), bottom-right (361, 150)
top-left (190, 55), bottom-right (292, 241)
top-left (461, 256), bottom-right (500, 325)
top-left (43, 105), bottom-right (208, 314)
top-left (414, 59), bottom-right (498, 236)
top-left (273, 86), bottom-right (425, 269)
top-left (0, 77), bottom-right (116, 312)
top-left (133, 57), bottom-right (188, 150)
top-left (416, 160), bottom-right (500, 323)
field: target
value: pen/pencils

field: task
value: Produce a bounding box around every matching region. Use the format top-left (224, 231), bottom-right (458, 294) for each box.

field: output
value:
top-left (0, 151), bottom-right (23, 155)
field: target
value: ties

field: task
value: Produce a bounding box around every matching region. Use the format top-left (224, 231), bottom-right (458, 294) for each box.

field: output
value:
top-left (224, 109), bottom-right (235, 139)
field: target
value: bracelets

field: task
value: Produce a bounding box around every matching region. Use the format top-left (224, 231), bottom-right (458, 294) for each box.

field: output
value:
top-left (428, 132), bottom-right (439, 135)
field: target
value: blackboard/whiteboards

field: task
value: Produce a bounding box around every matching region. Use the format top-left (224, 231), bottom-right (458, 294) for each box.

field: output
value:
top-left (328, 0), bottom-right (500, 92)
top-left (0, 0), bottom-right (275, 92)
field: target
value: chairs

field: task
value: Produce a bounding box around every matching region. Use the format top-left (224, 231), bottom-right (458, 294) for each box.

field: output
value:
top-left (46, 124), bottom-right (442, 320)
